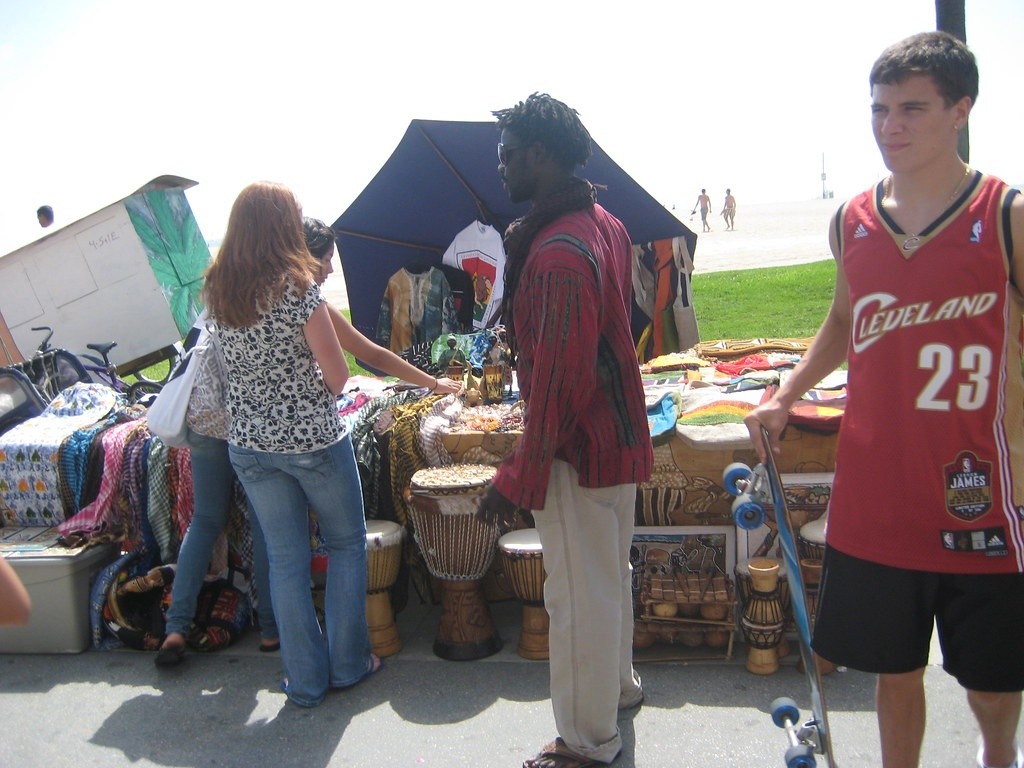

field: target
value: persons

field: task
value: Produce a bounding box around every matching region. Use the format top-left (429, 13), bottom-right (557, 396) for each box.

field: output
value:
top-left (722, 188), bottom-right (737, 229)
top-left (0, 549), bottom-right (31, 628)
top-left (744, 28), bottom-right (1024, 768)
top-left (155, 216), bottom-right (462, 668)
top-left (474, 91), bottom-right (654, 768)
top-left (200, 179), bottom-right (383, 710)
top-left (693, 188), bottom-right (712, 231)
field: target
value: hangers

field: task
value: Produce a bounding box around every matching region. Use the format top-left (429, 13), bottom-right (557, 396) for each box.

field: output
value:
top-left (477, 206), bottom-right (492, 226)
top-left (405, 247), bottom-right (434, 269)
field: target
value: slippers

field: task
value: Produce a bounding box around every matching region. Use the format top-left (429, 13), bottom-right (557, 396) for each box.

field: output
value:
top-left (279, 677), bottom-right (290, 693)
top-left (260, 637), bottom-right (281, 652)
top-left (153, 632), bottom-right (186, 668)
top-left (342, 652), bottom-right (385, 691)
top-left (523, 737), bottom-right (623, 768)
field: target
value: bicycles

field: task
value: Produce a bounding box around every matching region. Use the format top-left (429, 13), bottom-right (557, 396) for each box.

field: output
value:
top-left (29, 325), bottom-right (164, 408)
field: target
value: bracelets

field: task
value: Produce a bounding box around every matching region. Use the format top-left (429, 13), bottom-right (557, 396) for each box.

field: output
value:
top-left (431, 377), bottom-right (437, 390)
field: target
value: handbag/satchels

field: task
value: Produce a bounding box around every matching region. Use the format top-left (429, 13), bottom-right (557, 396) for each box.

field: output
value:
top-left (146, 303), bottom-right (209, 449)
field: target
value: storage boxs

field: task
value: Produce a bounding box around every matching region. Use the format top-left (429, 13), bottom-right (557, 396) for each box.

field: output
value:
top-left (0, 543), bottom-right (114, 653)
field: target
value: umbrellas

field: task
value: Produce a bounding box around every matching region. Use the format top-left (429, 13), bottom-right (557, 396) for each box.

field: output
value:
top-left (327, 119), bottom-right (701, 376)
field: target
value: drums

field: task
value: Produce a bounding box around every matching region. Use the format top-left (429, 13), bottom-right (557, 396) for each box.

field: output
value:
top-left (733, 517), bottom-right (835, 677)
top-left (363, 518), bottom-right (406, 659)
top-left (406, 463), bottom-right (506, 663)
top-left (496, 526), bottom-right (549, 662)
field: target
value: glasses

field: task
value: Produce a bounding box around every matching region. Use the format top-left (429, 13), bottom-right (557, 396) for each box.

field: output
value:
top-left (497, 142), bottom-right (543, 167)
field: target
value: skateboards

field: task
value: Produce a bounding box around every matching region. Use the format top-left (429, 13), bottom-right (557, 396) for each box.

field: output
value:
top-left (723, 423), bottom-right (837, 768)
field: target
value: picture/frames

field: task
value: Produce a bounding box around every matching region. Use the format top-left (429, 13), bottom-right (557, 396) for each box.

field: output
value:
top-left (737, 471), bottom-right (836, 640)
top-left (630, 525), bottom-right (737, 610)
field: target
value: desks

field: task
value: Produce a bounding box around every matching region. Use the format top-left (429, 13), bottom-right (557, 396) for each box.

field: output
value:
top-left (441, 429), bottom-right (837, 633)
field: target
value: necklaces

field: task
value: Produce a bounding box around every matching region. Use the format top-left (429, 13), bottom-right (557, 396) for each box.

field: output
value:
top-left (881, 163), bottom-right (971, 237)
